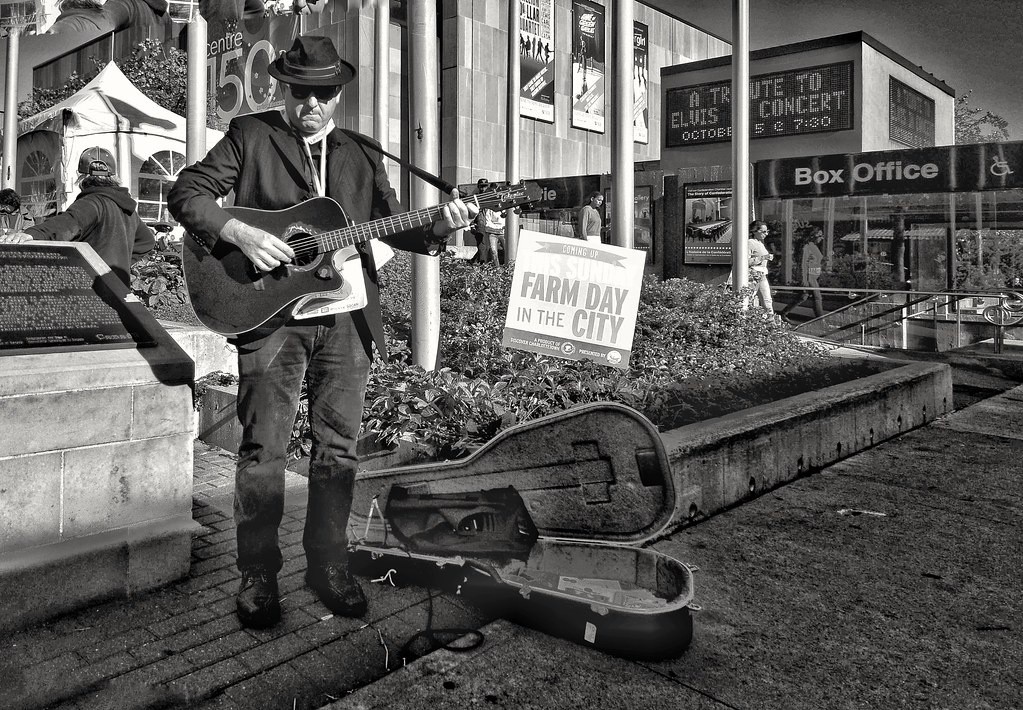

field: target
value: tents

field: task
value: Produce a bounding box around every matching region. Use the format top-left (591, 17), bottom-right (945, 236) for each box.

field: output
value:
top-left (14, 29), bottom-right (230, 239)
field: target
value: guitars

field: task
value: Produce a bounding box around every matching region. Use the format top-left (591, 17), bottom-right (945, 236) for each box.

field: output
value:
top-left (180, 178), bottom-right (548, 346)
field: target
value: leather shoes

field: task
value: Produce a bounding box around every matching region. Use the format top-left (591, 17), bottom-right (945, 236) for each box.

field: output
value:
top-left (237, 570), bottom-right (280, 627)
top-left (305, 561), bottom-right (366, 615)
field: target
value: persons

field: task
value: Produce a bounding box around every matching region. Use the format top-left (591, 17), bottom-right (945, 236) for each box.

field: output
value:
top-left (519, 33), bottom-right (555, 66)
top-left (577, 35), bottom-right (588, 72)
top-left (469, 178), bottom-right (491, 265)
top-left (578, 191), bottom-right (604, 243)
top-left (166, 34), bottom-right (480, 630)
top-left (485, 183), bottom-right (506, 266)
top-left (0, 146), bottom-right (155, 289)
top-left (777, 226), bottom-right (826, 325)
top-left (747, 220), bottom-right (778, 330)
top-left (0, 188), bottom-right (34, 237)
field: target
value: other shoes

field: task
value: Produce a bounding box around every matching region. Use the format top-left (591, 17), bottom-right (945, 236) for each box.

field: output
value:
top-left (779, 313), bottom-right (792, 323)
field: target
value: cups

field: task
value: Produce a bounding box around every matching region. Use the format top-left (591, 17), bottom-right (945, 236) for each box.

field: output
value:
top-left (769, 254), bottom-right (773, 260)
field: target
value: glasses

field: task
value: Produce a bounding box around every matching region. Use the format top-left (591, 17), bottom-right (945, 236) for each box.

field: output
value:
top-left (757, 230), bottom-right (770, 234)
top-left (285, 82), bottom-right (335, 101)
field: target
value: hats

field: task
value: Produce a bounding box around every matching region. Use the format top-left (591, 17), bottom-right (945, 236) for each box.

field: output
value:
top-left (478, 179), bottom-right (489, 186)
top-left (268, 35), bottom-right (356, 87)
top-left (78, 146), bottom-right (116, 175)
